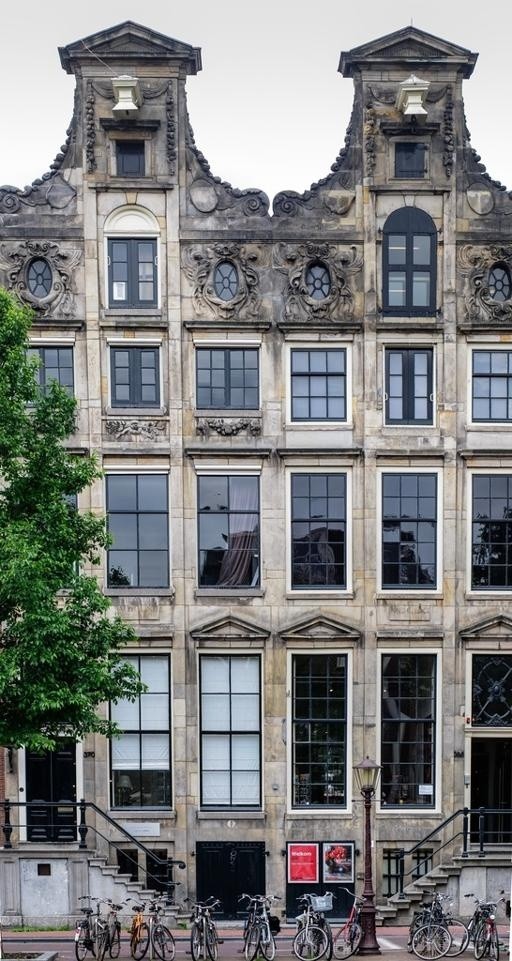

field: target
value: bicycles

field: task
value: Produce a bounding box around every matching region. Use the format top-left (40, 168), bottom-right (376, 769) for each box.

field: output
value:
top-left (183, 895), bottom-right (223, 961)
top-left (73, 895), bottom-right (176, 961)
top-left (292, 887), bottom-right (367, 961)
top-left (407, 890), bottom-right (509, 961)
top-left (237, 893), bottom-right (282, 961)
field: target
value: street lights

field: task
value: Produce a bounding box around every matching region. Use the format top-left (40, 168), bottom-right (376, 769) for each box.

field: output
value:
top-left (351, 754), bottom-right (383, 956)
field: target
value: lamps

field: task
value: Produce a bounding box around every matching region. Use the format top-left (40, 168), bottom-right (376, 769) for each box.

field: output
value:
top-left (395, 72), bottom-right (431, 122)
top-left (117, 776), bottom-right (133, 806)
top-left (110, 74), bottom-right (143, 120)
